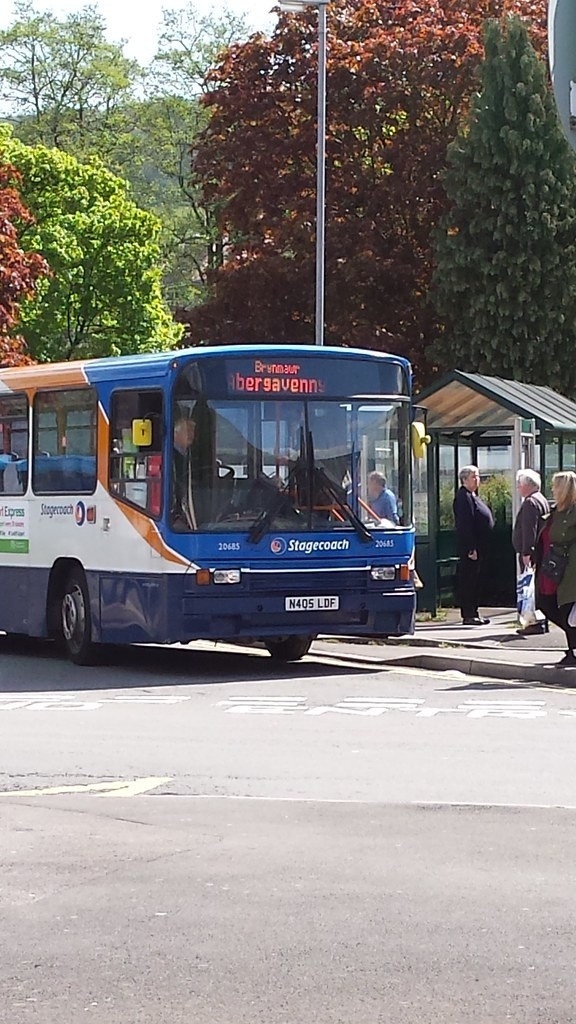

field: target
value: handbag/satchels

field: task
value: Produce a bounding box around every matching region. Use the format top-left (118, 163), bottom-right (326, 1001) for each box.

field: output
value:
top-left (517, 563), bottom-right (537, 623)
top-left (539, 549), bottom-right (568, 585)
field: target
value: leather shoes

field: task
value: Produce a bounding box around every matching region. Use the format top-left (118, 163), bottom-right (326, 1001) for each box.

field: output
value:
top-left (463, 617), bottom-right (490, 625)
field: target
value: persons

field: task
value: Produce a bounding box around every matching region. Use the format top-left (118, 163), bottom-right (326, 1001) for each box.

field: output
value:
top-left (453, 465), bottom-right (492, 625)
top-left (512, 470), bottom-right (549, 636)
top-left (285, 402), bottom-right (348, 519)
top-left (368, 471), bottom-right (400, 525)
top-left (169, 418), bottom-right (213, 531)
top-left (530, 473), bottom-right (576, 668)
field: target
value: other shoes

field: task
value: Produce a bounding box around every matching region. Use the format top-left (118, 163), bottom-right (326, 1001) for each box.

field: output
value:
top-left (556, 650), bottom-right (576, 667)
top-left (516, 622), bottom-right (544, 634)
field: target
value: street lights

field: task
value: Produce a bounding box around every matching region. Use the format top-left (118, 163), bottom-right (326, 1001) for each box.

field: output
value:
top-left (276, 0), bottom-right (328, 348)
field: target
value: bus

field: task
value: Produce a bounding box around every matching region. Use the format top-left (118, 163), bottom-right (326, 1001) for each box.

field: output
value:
top-left (0, 344), bottom-right (431, 672)
